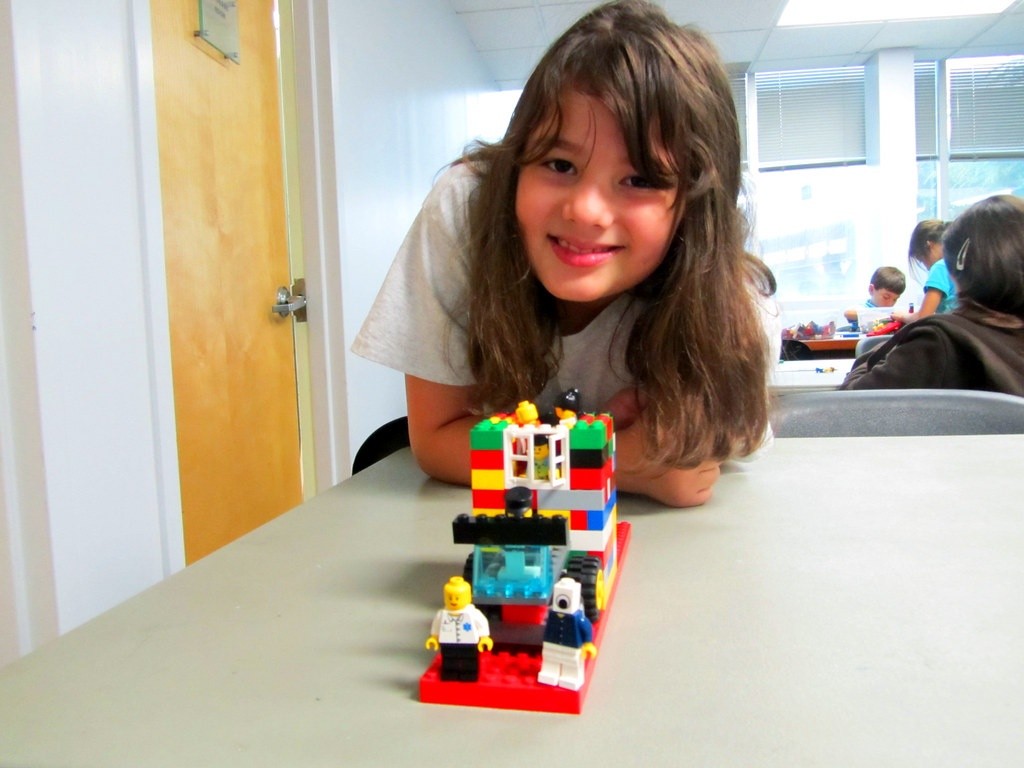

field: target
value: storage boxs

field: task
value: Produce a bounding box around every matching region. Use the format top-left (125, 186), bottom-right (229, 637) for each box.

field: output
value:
top-left (858, 307), bottom-right (901, 334)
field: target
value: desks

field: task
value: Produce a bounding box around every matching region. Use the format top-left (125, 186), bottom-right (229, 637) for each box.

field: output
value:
top-left (766, 360), bottom-right (857, 395)
top-left (0, 435), bottom-right (1024, 768)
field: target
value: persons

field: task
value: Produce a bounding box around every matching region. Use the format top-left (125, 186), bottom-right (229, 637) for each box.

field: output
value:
top-left (840, 195), bottom-right (1024, 400)
top-left (347, 0), bottom-right (782, 509)
top-left (892, 220), bottom-right (960, 324)
top-left (843, 266), bottom-right (906, 322)
